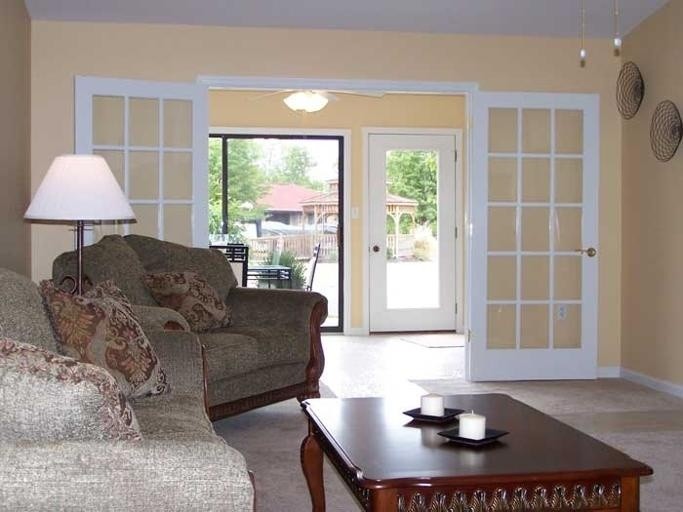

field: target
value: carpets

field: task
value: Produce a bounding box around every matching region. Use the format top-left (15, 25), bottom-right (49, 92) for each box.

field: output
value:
top-left (400, 332), bottom-right (463, 348)
top-left (213, 378), bottom-right (371, 511)
top-left (581, 431), bottom-right (682, 512)
top-left (409, 375), bottom-right (682, 414)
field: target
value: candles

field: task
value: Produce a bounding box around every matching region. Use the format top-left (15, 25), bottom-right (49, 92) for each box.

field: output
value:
top-left (420, 393), bottom-right (445, 417)
top-left (458, 407), bottom-right (486, 440)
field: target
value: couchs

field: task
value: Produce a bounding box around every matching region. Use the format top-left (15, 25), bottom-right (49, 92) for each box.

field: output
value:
top-left (0, 263), bottom-right (254, 512)
top-left (47, 233), bottom-right (328, 421)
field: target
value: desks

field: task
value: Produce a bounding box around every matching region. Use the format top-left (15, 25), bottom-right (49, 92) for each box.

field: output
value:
top-left (247, 264), bottom-right (291, 289)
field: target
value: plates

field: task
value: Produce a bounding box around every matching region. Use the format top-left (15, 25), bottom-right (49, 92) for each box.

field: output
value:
top-left (401, 405), bottom-right (464, 421)
top-left (437, 424), bottom-right (509, 446)
top-left (402, 417), bottom-right (463, 431)
top-left (439, 440), bottom-right (507, 453)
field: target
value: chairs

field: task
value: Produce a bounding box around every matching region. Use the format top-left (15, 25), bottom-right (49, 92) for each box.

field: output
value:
top-left (209, 246), bottom-right (249, 288)
top-left (301, 243), bottom-right (322, 290)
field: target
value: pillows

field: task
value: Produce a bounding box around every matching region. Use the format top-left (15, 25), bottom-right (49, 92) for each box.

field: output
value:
top-left (1, 333), bottom-right (140, 446)
top-left (139, 264), bottom-right (234, 333)
top-left (41, 276), bottom-right (171, 397)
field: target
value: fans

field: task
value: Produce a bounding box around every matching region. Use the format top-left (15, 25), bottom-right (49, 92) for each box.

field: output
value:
top-left (253, 87), bottom-right (388, 102)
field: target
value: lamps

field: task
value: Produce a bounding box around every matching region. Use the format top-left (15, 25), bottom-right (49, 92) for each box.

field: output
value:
top-left (19, 153), bottom-right (135, 299)
top-left (282, 91), bottom-right (327, 115)
